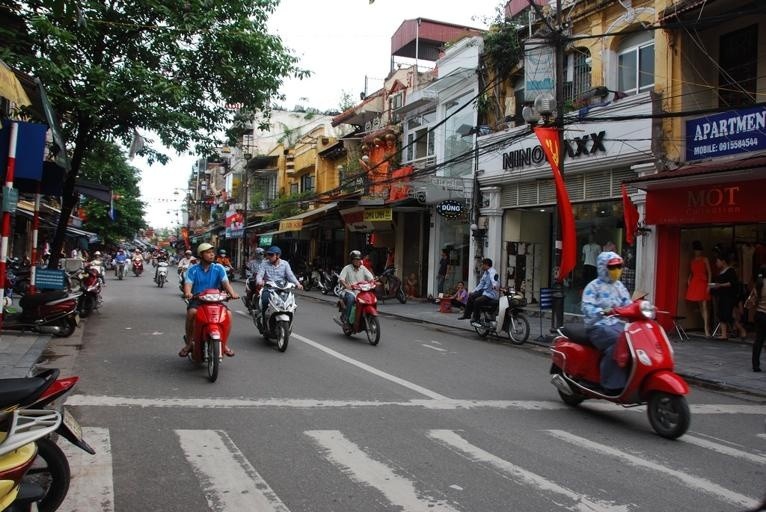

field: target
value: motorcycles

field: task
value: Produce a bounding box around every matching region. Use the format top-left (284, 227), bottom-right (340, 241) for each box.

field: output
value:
top-left (470, 286), bottom-right (529, 346)
top-left (153, 261), bottom-right (169, 288)
top-left (132, 258), bottom-right (144, 277)
top-left (1, 289), bottom-right (83, 337)
top-left (549, 302), bottom-right (691, 439)
top-left (115, 260), bottom-right (128, 280)
top-left (1, 368), bottom-right (96, 511)
top-left (177, 265), bottom-right (192, 293)
top-left (333, 275), bottom-right (382, 347)
top-left (183, 289), bottom-right (242, 383)
top-left (0, 408), bottom-right (62, 512)
top-left (78, 263), bottom-right (104, 319)
top-left (248, 278), bottom-right (304, 353)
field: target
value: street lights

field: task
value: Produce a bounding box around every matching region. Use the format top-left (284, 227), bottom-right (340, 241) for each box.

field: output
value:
top-left (523, 91), bottom-right (570, 333)
top-left (166, 186), bottom-right (198, 238)
top-left (221, 137), bottom-right (257, 280)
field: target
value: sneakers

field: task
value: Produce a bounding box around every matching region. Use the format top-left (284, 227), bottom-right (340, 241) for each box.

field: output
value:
top-left (458, 314), bottom-right (472, 320)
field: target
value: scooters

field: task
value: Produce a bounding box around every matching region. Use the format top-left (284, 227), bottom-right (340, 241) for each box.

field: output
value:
top-left (295, 258), bottom-right (342, 295)
top-left (375, 264), bottom-right (407, 304)
top-left (142, 250), bottom-right (177, 268)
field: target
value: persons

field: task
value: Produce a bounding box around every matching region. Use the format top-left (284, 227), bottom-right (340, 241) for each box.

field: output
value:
top-left (581, 250), bottom-right (633, 392)
top-left (686, 246), bottom-right (711, 336)
top-left (256, 246), bottom-right (303, 326)
top-left (288, 256), bottom-right (295, 274)
top-left (580, 234), bottom-right (601, 283)
top-left (246, 248), bottom-right (265, 304)
top-left (446, 281), bottom-right (468, 311)
top-left (216, 250), bottom-right (233, 269)
top-left (437, 248), bottom-right (448, 291)
top-left (177, 250), bottom-right (195, 287)
top-left (363, 254), bottom-right (373, 271)
top-left (116, 247), bottom-right (143, 274)
top-left (176, 242), bottom-right (235, 357)
top-left (458, 258), bottom-right (500, 322)
top-left (339, 249), bottom-right (374, 323)
top-left (143, 248), bottom-right (169, 281)
top-left (70, 246), bottom-right (114, 286)
top-left (387, 248), bottom-right (395, 267)
top-left (751, 270), bottom-right (766, 372)
top-left (712, 253), bottom-right (748, 340)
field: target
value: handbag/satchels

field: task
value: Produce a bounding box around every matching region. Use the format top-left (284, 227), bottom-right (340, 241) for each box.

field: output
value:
top-left (744, 286), bottom-right (759, 311)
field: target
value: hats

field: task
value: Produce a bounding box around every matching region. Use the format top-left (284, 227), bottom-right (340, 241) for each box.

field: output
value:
top-left (608, 258), bottom-right (624, 265)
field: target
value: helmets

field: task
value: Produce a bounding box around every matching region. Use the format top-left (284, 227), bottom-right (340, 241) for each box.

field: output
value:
top-left (219, 249), bottom-right (227, 254)
top-left (196, 242), bottom-right (215, 258)
top-left (185, 250), bottom-right (192, 255)
top-left (265, 245), bottom-right (282, 254)
top-left (255, 247), bottom-right (265, 257)
top-left (349, 249), bottom-right (362, 259)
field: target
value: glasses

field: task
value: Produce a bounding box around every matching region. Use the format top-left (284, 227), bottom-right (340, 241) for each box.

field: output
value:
top-left (607, 263), bottom-right (622, 270)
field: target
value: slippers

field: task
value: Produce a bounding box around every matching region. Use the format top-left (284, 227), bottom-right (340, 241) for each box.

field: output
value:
top-left (179, 345), bottom-right (193, 357)
top-left (224, 349), bottom-right (235, 357)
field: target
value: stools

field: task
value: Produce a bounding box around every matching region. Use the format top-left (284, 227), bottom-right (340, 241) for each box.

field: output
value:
top-left (664, 314), bottom-right (691, 346)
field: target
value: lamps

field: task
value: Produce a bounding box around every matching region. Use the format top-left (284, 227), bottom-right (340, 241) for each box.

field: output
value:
top-left (470, 221), bottom-right (489, 239)
